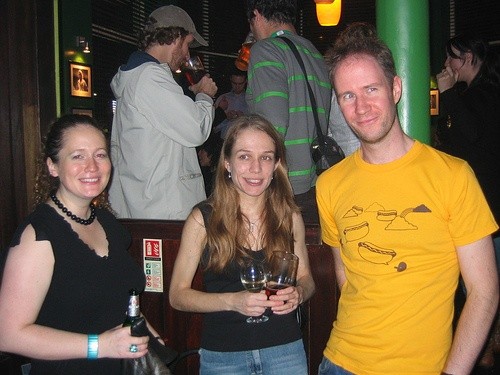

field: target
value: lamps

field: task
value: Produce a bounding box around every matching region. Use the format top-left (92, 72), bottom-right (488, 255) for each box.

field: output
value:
top-left (77, 35), bottom-right (91, 54)
top-left (314, 0), bottom-right (341, 28)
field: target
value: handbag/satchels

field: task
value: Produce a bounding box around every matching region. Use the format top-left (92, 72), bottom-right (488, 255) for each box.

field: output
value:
top-left (310, 133), bottom-right (346, 173)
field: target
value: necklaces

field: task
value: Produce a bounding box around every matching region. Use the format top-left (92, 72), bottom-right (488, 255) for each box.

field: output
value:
top-left (51, 190), bottom-right (96, 226)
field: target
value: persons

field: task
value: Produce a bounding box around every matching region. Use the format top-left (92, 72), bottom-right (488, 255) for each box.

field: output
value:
top-left (246, 0), bottom-right (330, 221)
top-left (169, 114), bottom-right (316, 375)
top-left (197, 70), bottom-right (248, 197)
top-left (0, 114), bottom-right (150, 375)
top-left (316, 37), bottom-right (500, 375)
top-left (107, 5), bottom-right (217, 221)
top-left (436, 30), bottom-right (500, 269)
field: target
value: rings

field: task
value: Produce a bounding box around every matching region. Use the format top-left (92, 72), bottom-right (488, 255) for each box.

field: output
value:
top-left (130, 345), bottom-right (137, 352)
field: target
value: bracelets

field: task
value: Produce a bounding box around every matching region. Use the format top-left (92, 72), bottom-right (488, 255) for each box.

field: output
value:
top-left (88, 334), bottom-right (98, 358)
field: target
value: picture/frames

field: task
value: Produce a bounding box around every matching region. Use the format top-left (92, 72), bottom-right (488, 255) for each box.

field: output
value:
top-left (68, 59), bottom-right (94, 99)
top-left (71, 106), bottom-right (94, 119)
top-left (430, 88), bottom-right (440, 116)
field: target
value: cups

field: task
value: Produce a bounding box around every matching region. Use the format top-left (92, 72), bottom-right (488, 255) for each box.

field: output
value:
top-left (234, 31), bottom-right (257, 72)
top-left (265, 250), bottom-right (299, 304)
top-left (183, 55), bottom-right (205, 86)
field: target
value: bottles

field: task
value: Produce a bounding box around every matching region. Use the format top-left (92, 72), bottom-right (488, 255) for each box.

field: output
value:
top-left (121, 287), bottom-right (141, 329)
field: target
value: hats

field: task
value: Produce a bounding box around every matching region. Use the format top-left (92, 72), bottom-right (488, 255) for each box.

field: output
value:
top-left (145, 4), bottom-right (209, 48)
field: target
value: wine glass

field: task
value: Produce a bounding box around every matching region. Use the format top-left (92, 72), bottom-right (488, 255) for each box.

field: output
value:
top-left (241, 260), bottom-right (270, 324)
